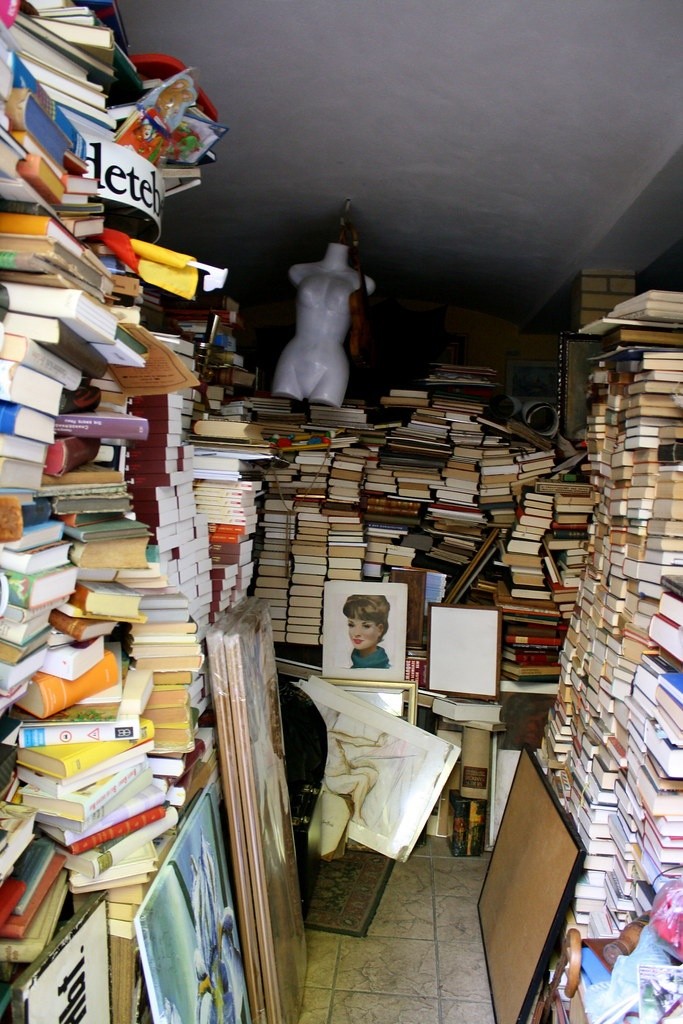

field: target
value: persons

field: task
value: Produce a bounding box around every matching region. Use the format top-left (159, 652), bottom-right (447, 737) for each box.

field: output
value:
top-left (270, 243), bottom-right (376, 408)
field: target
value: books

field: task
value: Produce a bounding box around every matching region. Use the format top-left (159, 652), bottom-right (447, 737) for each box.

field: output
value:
top-left (416, 696), bottom-right (521, 857)
top-left (0, 0), bottom-right (275, 963)
top-left (532, 287), bottom-right (683, 1024)
top-left (248, 364), bottom-right (595, 695)
